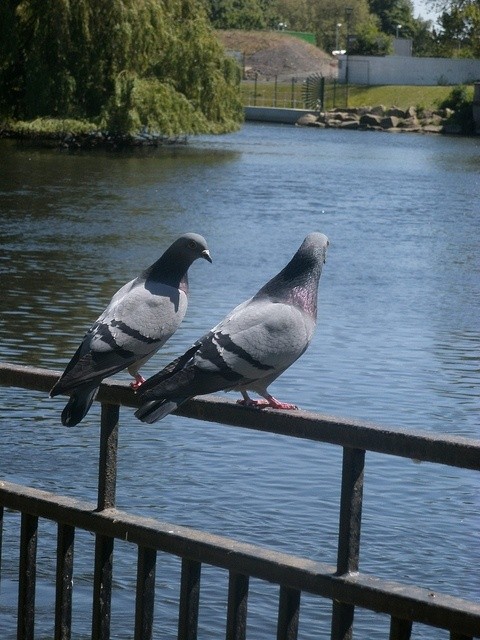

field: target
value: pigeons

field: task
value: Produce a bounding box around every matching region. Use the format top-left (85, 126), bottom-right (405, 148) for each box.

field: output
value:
top-left (47, 231), bottom-right (213, 428)
top-left (134, 230), bottom-right (329, 422)
